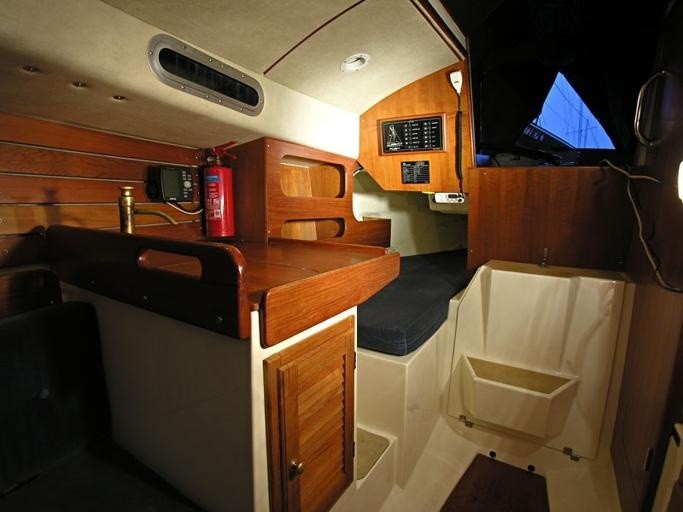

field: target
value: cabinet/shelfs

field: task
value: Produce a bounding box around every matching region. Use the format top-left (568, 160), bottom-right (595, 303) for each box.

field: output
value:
top-left (76, 265), bottom-right (357, 511)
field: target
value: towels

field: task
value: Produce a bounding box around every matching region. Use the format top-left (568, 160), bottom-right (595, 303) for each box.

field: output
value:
top-left (431, 452), bottom-right (548, 511)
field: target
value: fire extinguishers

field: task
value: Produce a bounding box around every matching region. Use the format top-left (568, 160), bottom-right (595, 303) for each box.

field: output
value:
top-left (204, 140), bottom-right (239, 242)
